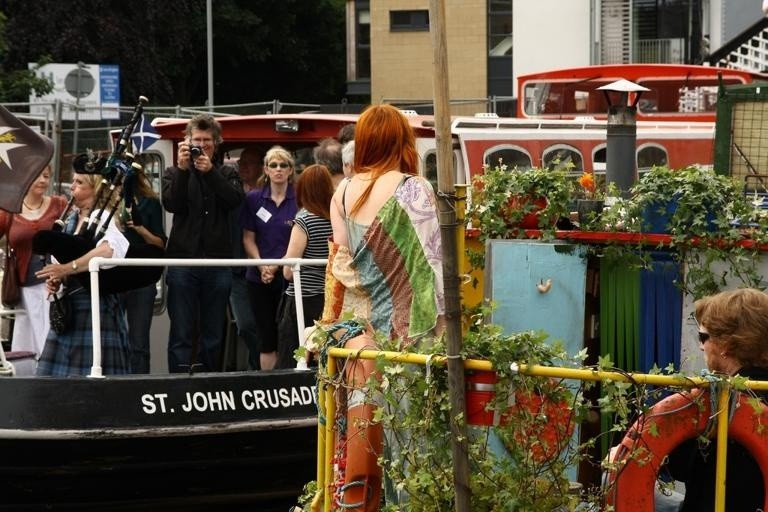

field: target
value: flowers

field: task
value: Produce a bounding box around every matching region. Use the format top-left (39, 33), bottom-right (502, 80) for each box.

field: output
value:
top-left (574, 169), bottom-right (601, 196)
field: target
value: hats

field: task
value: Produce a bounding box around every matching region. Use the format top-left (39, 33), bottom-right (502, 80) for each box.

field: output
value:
top-left (73, 153), bottom-right (117, 178)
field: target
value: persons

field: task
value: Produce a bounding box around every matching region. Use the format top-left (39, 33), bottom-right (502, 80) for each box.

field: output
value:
top-left (158, 111), bottom-right (245, 374)
top-left (336, 122), bottom-right (356, 144)
top-left (312, 136), bottom-right (346, 187)
top-left (326, 101), bottom-right (446, 508)
top-left (0, 153), bottom-right (70, 358)
top-left (112, 155), bottom-right (167, 374)
top-left (32, 154), bottom-right (134, 377)
top-left (339, 139), bottom-right (357, 177)
top-left (280, 161), bottom-right (337, 370)
top-left (240, 144), bottom-right (303, 373)
top-left (598, 287), bottom-right (767, 512)
top-left (231, 142), bottom-right (263, 372)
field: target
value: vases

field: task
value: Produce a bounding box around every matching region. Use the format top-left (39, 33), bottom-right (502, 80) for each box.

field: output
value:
top-left (576, 198), bottom-right (607, 228)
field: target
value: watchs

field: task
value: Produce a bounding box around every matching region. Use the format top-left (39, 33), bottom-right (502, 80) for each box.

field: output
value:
top-left (71, 258), bottom-right (79, 274)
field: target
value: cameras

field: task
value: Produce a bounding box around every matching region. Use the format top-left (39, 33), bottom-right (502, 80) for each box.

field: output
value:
top-left (190, 145), bottom-right (202, 158)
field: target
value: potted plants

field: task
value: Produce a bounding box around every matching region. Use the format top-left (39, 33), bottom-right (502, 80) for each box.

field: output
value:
top-left (290, 297), bottom-right (764, 512)
top-left (459, 156), bottom-right (574, 270)
top-left (583, 164), bottom-right (763, 327)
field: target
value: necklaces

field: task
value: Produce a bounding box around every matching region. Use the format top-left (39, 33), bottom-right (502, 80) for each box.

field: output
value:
top-left (22, 199), bottom-right (45, 213)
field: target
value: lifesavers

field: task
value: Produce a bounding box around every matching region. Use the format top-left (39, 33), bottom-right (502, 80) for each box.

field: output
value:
top-left (607, 388), bottom-right (768, 511)
top-left (330, 319), bottom-right (384, 512)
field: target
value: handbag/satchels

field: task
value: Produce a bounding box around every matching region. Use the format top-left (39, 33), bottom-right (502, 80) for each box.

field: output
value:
top-left (0, 251), bottom-right (22, 308)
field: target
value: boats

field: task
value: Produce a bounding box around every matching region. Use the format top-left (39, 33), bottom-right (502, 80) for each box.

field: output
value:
top-left (0, 63), bottom-right (768, 512)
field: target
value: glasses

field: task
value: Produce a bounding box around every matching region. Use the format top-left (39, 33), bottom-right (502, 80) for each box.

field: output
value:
top-left (697, 331), bottom-right (710, 344)
